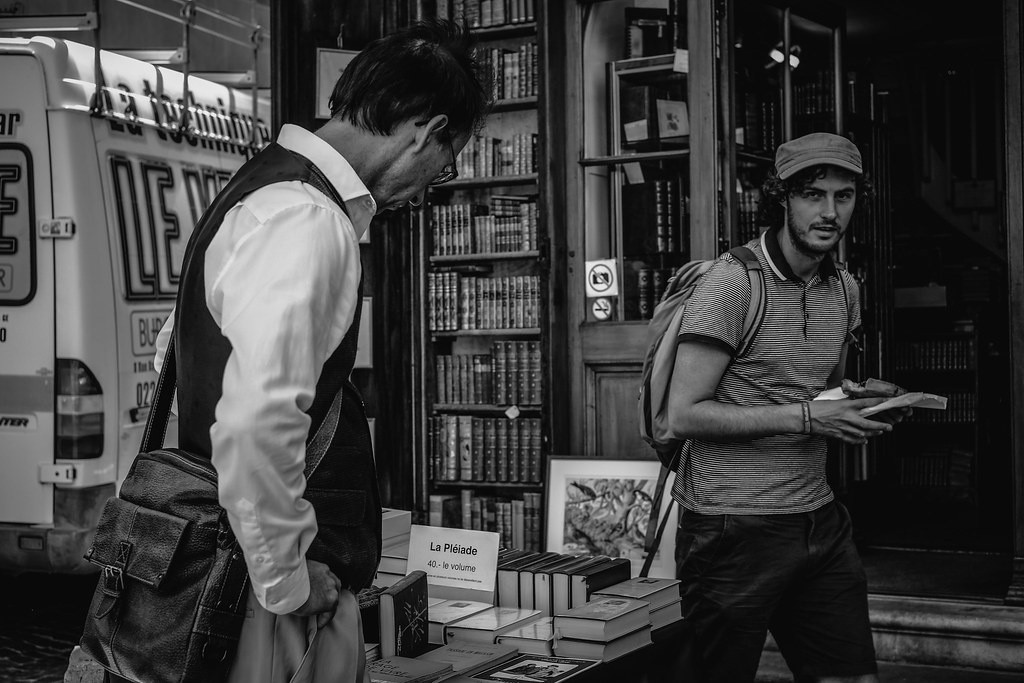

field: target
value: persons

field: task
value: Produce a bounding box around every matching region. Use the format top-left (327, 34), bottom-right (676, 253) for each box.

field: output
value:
top-left (668, 133), bottom-right (914, 683)
top-left (153, 16), bottom-right (493, 683)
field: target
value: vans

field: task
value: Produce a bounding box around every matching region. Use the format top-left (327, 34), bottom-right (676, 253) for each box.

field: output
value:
top-left (0, 35), bottom-right (272, 582)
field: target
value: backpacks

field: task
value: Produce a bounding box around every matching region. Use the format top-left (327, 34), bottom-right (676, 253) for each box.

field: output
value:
top-left (637, 242), bottom-right (848, 473)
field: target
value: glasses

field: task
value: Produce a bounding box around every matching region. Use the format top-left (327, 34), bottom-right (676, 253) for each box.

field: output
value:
top-left (415, 120), bottom-right (460, 186)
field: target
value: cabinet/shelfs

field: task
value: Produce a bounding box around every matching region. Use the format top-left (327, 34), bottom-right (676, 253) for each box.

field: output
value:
top-left (269, 1), bottom-right (841, 551)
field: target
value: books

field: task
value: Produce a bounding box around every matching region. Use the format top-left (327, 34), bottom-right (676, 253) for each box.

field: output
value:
top-left (333, 0), bottom-right (979, 683)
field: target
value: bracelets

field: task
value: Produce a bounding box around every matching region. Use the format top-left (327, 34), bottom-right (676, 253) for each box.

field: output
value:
top-left (801, 402), bottom-right (812, 435)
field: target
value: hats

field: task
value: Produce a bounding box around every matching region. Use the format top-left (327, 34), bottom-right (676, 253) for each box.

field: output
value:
top-left (774, 132), bottom-right (863, 181)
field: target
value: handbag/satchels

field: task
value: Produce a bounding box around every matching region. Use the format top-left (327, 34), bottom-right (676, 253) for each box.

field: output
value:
top-left (79, 448), bottom-right (254, 683)
